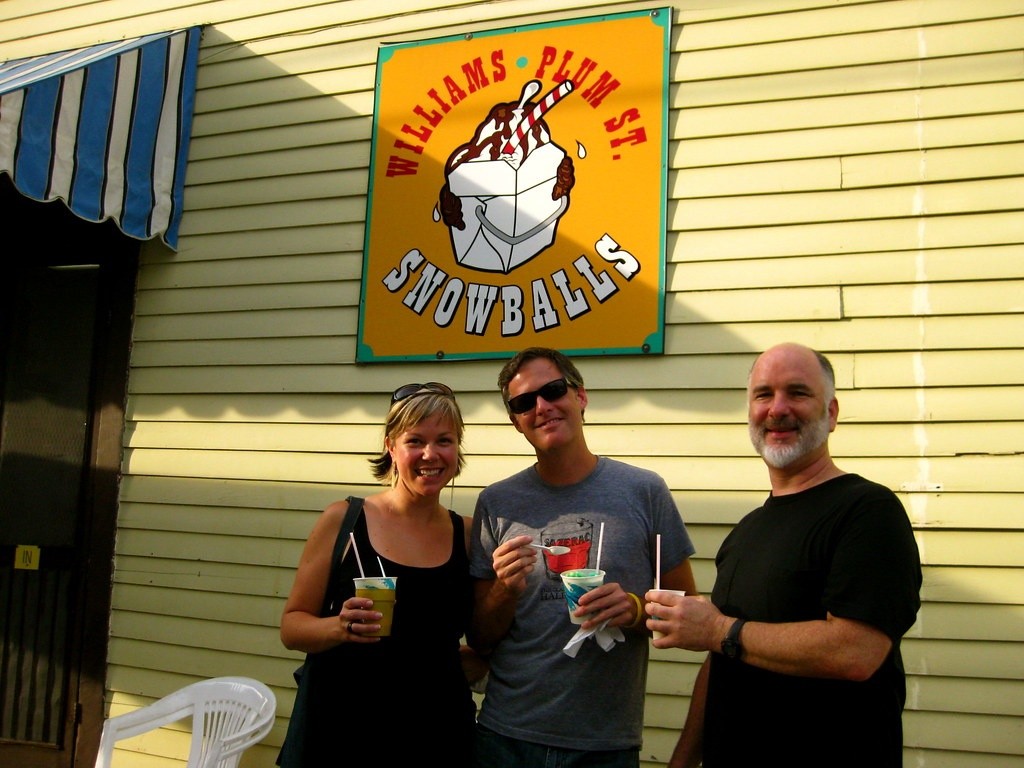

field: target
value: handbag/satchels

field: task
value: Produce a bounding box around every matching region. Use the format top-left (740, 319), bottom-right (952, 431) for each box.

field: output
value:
top-left (275, 496), bottom-right (365, 768)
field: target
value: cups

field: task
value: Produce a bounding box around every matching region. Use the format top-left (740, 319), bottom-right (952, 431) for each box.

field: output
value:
top-left (353, 577), bottom-right (398, 637)
top-left (560, 568), bottom-right (605, 624)
top-left (649, 589), bottom-right (685, 640)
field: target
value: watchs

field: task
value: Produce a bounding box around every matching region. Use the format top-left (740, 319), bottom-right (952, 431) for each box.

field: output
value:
top-left (719, 617), bottom-right (748, 665)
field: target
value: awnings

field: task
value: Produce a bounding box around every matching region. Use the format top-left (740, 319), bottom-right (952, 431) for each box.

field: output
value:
top-left (0, 27), bottom-right (203, 251)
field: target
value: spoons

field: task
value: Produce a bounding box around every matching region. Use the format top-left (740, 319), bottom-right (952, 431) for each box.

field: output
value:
top-left (529, 543), bottom-right (571, 555)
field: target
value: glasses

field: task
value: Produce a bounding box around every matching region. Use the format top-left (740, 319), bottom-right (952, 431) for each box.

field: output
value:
top-left (507, 377), bottom-right (577, 415)
top-left (390, 382), bottom-right (456, 405)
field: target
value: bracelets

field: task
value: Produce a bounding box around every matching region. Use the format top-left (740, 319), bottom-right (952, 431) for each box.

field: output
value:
top-left (624, 592), bottom-right (641, 629)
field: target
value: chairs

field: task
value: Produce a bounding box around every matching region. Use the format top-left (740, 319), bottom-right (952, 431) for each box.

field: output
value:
top-left (94, 674), bottom-right (278, 768)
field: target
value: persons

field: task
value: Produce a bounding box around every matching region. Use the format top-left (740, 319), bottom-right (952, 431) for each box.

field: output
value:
top-left (276, 381), bottom-right (496, 768)
top-left (644, 341), bottom-right (924, 768)
top-left (464, 348), bottom-right (697, 768)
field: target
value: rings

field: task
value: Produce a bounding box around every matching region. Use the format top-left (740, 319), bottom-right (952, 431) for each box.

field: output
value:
top-left (347, 620), bottom-right (355, 631)
